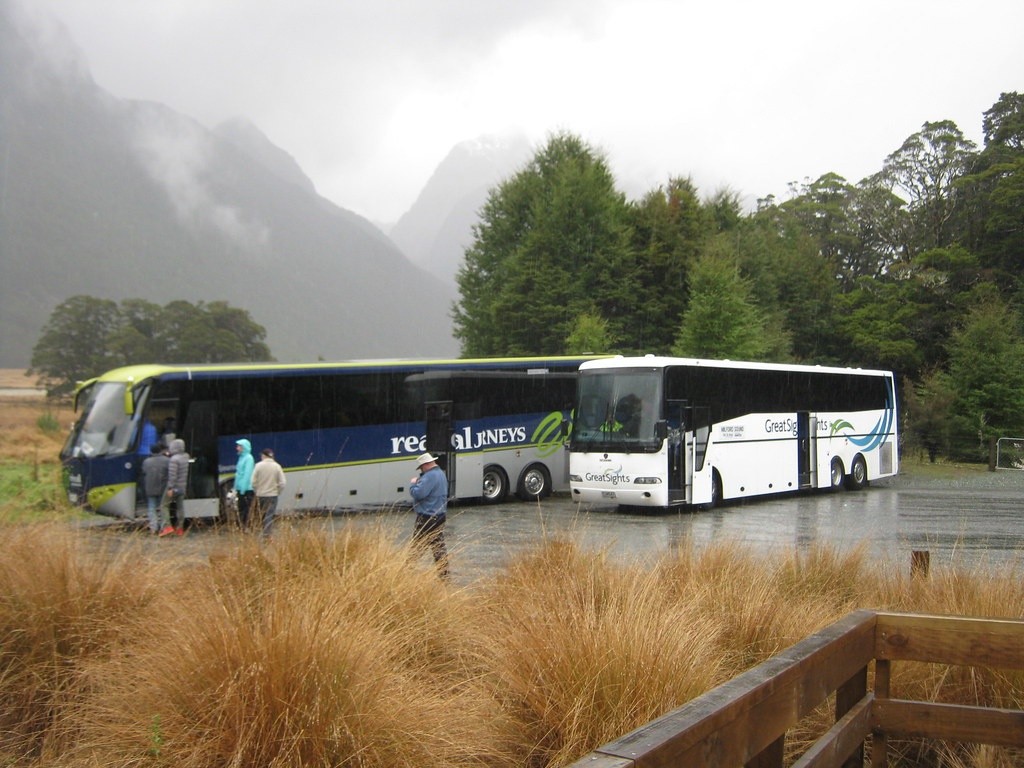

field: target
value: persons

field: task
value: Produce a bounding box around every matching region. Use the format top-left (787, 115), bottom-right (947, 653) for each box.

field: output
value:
top-left (232, 438), bottom-right (255, 532)
top-left (599, 413), bottom-right (624, 432)
top-left (137, 418), bottom-right (189, 538)
top-left (409, 452), bottom-right (450, 584)
top-left (252, 448), bottom-right (286, 537)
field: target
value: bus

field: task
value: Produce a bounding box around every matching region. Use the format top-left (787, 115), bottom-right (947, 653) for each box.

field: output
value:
top-left (61, 356), bottom-right (623, 532)
top-left (570, 359), bottom-right (898, 508)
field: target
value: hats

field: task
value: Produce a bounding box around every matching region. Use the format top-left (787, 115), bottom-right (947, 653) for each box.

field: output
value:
top-left (259, 448), bottom-right (274, 458)
top-left (150, 445), bottom-right (161, 453)
top-left (415, 452), bottom-right (438, 470)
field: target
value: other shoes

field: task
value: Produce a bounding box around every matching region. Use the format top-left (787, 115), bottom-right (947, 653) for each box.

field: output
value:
top-left (172, 528), bottom-right (183, 536)
top-left (159, 525), bottom-right (174, 537)
